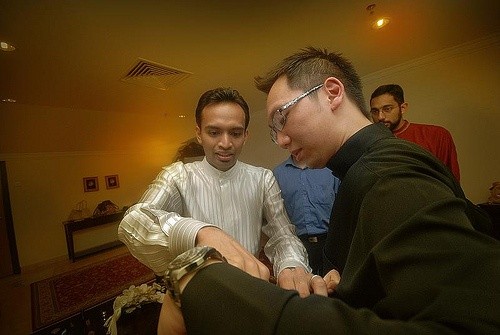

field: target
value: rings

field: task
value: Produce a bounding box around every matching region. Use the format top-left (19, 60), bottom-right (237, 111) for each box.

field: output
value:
top-left (311, 274), bottom-right (322, 285)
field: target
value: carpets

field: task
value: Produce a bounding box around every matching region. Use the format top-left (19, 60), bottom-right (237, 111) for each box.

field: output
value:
top-left (30, 251), bottom-right (161, 333)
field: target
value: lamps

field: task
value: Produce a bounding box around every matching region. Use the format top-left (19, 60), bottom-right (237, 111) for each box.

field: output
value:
top-left (366, 3), bottom-right (392, 31)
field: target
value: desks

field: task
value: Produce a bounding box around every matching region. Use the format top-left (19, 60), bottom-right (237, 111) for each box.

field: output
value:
top-left (62, 210), bottom-right (127, 264)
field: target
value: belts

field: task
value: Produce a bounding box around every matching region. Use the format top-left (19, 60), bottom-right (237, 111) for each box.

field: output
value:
top-left (299, 234), bottom-right (327, 244)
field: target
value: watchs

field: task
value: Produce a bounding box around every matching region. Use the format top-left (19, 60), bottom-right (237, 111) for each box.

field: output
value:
top-left (164, 246), bottom-right (227, 306)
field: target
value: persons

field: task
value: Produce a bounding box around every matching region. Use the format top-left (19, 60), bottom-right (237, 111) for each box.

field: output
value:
top-left (272, 155), bottom-right (341, 279)
top-left (369, 83), bottom-right (461, 184)
top-left (156, 44), bottom-right (500, 335)
top-left (118, 87), bottom-right (327, 299)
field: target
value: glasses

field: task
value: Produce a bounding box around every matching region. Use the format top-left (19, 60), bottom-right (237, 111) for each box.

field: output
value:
top-left (269, 83), bottom-right (325, 144)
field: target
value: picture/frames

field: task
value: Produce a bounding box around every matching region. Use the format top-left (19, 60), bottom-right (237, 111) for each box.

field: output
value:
top-left (105, 175), bottom-right (121, 190)
top-left (83, 176), bottom-right (99, 193)
